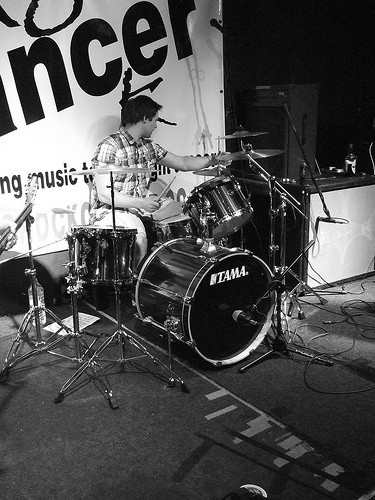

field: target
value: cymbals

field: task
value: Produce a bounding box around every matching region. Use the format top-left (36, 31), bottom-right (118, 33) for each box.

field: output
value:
top-left (60, 167), bottom-right (160, 175)
top-left (215, 131), bottom-right (268, 139)
top-left (193, 169), bottom-right (244, 176)
top-left (215, 149), bottom-right (284, 160)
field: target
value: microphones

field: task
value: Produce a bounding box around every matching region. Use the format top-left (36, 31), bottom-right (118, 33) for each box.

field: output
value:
top-left (210, 19), bottom-right (225, 34)
top-left (316, 216), bottom-right (340, 223)
top-left (232, 309), bottom-right (258, 326)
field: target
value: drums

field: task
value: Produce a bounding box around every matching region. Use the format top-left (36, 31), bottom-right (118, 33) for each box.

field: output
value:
top-left (158, 218), bottom-right (194, 243)
top-left (134, 238), bottom-right (277, 366)
top-left (181, 176), bottom-right (255, 243)
top-left (67, 225), bottom-right (138, 285)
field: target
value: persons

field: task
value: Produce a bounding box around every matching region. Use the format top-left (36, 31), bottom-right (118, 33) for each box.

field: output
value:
top-left (88, 95), bottom-right (232, 308)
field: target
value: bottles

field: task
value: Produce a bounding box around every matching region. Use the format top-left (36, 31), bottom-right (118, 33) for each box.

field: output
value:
top-left (28, 278), bottom-right (47, 327)
top-left (344, 146), bottom-right (356, 175)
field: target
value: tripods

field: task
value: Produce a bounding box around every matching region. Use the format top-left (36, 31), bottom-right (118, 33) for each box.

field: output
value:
top-left (238, 139), bottom-right (335, 373)
top-left (0, 172), bottom-right (190, 410)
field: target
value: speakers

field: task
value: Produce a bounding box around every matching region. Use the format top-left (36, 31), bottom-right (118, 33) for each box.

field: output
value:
top-left (239, 172), bottom-right (375, 295)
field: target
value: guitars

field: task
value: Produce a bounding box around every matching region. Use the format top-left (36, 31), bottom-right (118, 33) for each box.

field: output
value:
top-left (0, 173), bottom-right (38, 256)
